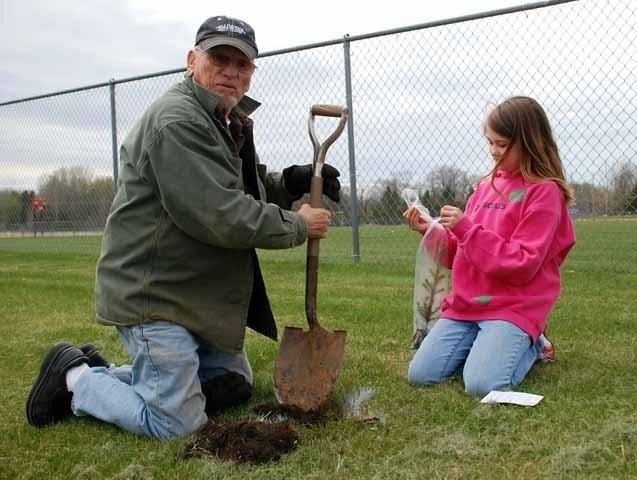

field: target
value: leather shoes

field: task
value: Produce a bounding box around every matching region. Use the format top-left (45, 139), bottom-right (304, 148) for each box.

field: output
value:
top-left (27, 343), bottom-right (108, 426)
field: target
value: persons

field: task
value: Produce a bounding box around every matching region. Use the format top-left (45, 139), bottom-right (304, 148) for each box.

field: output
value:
top-left (24, 14), bottom-right (340, 440)
top-left (400, 95), bottom-right (577, 398)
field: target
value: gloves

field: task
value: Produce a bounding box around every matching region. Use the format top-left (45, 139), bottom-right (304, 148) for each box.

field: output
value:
top-left (284, 164), bottom-right (341, 203)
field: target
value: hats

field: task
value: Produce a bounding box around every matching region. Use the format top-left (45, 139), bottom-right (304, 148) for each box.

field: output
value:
top-left (194, 15), bottom-right (257, 62)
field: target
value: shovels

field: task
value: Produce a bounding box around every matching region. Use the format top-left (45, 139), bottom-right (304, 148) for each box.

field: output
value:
top-left (273, 103), bottom-right (349, 413)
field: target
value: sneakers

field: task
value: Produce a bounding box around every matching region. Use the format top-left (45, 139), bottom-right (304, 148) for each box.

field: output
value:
top-left (540, 334), bottom-right (554, 363)
